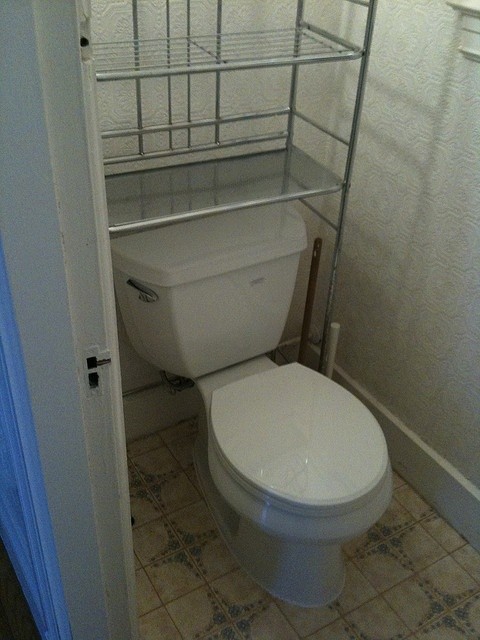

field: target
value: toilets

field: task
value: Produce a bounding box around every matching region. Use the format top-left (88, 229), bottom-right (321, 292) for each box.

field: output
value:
top-left (110, 199), bottom-right (394, 609)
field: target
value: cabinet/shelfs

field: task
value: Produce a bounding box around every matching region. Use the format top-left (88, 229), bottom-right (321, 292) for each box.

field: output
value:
top-left (90, 0), bottom-right (379, 399)
